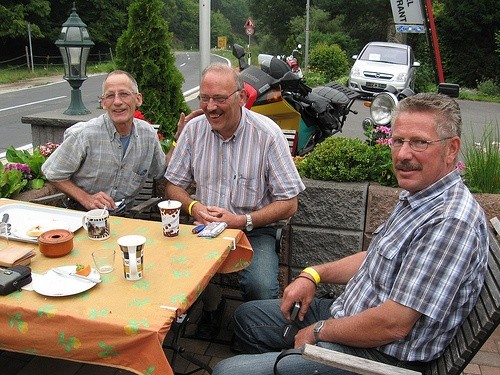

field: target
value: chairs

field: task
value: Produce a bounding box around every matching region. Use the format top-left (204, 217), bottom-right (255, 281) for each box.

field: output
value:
top-left (128, 130), bottom-right (300, 343)
top-left (273, 216), bottom-right (500, 375)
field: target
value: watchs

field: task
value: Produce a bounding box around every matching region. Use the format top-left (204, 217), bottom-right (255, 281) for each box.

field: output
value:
top-left (314, 319), bottom-right (325, 342)
top-left (246, 214), bottom-right (254, 231)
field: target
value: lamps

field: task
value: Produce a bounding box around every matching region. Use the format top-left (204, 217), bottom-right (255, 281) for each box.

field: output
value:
top-left (55, 2), bottom-right (94, 114)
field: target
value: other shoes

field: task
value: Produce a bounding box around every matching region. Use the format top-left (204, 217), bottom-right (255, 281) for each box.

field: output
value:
top-left (196, 297), bottom-right (227, 339)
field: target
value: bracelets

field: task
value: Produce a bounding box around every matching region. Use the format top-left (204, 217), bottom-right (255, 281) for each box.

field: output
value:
top-left (189, 200), bottom-right (197, 215)
top-left (172, 136), bottom-right (177, 142)
top-left (302, 268), bottom-right (320, 285)
top-left (172, 141), bottom-right (177, 147)
top-left (294, 275), bottom-right (317, 289)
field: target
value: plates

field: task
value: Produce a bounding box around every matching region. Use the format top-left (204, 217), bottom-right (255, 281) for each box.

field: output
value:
top-left (32, 265), bottom-right (101, 298)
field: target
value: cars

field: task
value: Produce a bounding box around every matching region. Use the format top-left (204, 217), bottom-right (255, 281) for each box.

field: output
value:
top-left (347, 42), bottom-right (421, 102)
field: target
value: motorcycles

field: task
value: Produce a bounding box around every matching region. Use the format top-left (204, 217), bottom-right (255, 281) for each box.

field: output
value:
top-left (232, 43), bottom-right (361, 157)
top-left (277, 43), bottom-right (304, 83)
top-left (361, 82), bottom-right (460, 133)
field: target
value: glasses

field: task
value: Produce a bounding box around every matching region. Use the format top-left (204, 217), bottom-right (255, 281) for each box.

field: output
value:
top-left (197, 88), bottom-right (239, 104)
top-left (387, 136), bottom-right (454, 152)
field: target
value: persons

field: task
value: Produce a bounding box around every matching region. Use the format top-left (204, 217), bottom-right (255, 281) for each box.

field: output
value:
top-left (41, 70), bottom-right (204, 217)
top-left (214, 92), bottom-right (490, 375)
top-left (163, 63), bottom-right (307, 301)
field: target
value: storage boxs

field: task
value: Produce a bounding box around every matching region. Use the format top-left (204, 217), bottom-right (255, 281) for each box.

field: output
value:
top-left (313, 86), bottom-right (350, 116)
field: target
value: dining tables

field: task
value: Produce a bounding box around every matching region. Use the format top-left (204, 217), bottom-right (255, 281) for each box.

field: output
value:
top-left (1, 197), bottom-right (243, 375)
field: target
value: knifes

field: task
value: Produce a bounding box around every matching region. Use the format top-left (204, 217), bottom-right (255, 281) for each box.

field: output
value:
top-left (51, 268), bottom-right (103, 285)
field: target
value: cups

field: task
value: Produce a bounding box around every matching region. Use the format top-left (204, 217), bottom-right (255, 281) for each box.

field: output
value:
top-left (91, 249), bottom-right (118, 273)
top-left (82, 209), bottom-right (111, 240)
top-left (117, 235), bottom-right (145, 281)
top-left (158, 200), bottom-right (183, 237)
